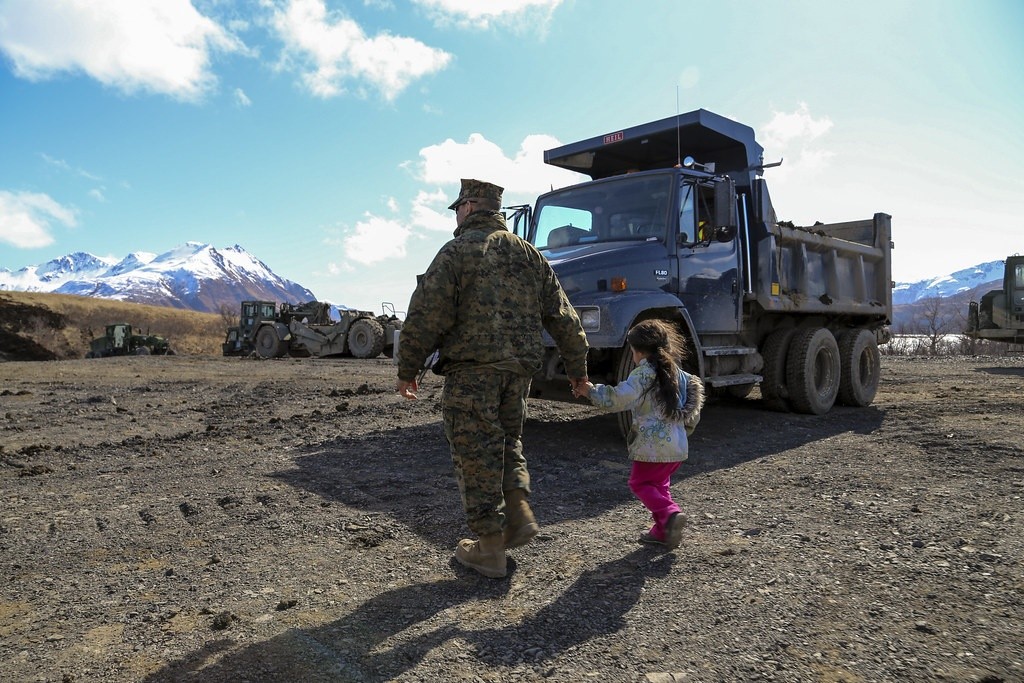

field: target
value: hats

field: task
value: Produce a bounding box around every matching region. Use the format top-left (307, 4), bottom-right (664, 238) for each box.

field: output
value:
top-left (448, 179), bottom-right (504, 209)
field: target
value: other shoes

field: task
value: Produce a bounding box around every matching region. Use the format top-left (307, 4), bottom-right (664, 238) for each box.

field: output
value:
top-left (640, 530), bottom-right (668, 546)
top-left (665, 512), bottom-right (687, 546)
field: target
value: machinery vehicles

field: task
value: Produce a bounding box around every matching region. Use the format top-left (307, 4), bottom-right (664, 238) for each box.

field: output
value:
top-left (84, 323), bottom-right (178, 358)
top-left (220, 299), bottom-right (408, 359)
top-left (962, 252), bottom-right (1024, 345)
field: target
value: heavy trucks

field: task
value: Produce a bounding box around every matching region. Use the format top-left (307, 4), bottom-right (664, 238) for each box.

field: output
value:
top-left (500, 107), bottom-right (897, 444)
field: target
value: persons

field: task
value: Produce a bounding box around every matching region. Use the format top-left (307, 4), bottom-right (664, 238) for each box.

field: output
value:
top-left (573, 319), bottom-right (703, 551)
top-left (395, 178), bottom-right (587, 579)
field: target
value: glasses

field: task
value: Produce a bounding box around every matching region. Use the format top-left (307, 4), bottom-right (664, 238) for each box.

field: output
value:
top-left (453, 201), bottom-right (477, 214)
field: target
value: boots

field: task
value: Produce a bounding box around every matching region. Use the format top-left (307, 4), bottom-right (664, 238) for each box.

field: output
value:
top-left (455, 534), bottom-right (507, 578)
top-left (502, 489), bottom-right (539, 549)
